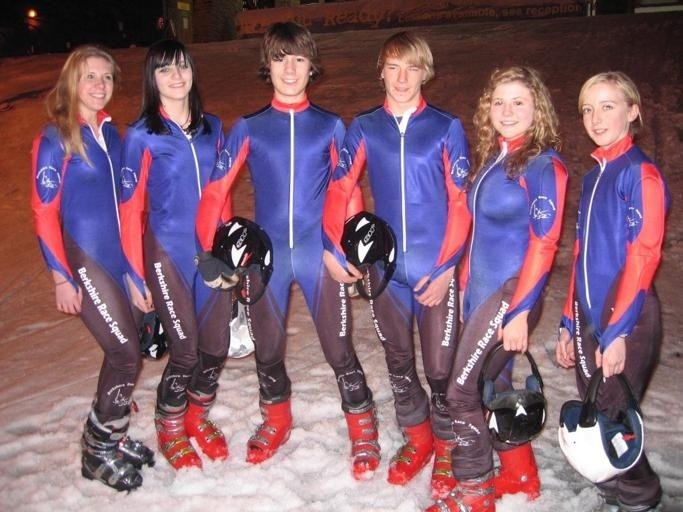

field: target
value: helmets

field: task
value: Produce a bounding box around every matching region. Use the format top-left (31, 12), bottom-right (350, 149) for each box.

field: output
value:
top-left (555, 362), bottom-right (646, 486)
top-left (208, 213), bottom-right (276, 307)
top-left (337, 209), bottom-right (399, 303)
top-left (135, 310), bottom-right (171, 362)
top-left (475, 340), bottom-right (549, 448)
top-left (220, 298), bottom-right (262, 360)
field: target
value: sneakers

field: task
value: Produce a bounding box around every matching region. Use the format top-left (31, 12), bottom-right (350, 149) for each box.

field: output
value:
top-left (597, 484), bottom-right (667, 512)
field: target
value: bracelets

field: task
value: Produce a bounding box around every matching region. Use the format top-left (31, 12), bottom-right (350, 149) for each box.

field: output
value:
top-left (55, 281), bottom-right (68, 286)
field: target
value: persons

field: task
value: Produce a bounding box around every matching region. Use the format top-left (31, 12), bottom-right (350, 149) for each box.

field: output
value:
top-left (556, 72), bottom-right (671, 512)
top-left (323, 32), bottom-right (470, 502)
top-left (120, 40), bottom-right (233, 472)
top-left (424, 66), bottom-right (568, 512)
top-left (32, 47), bottom-right (154, 489)
top-left (195, 21), bottom-right (382, 481)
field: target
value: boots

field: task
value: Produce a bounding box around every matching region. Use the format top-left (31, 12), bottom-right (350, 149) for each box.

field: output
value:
top-left (150, 395), bottom-right (204, 477)
top-left (242, 393), bottom-right (296, 469)
top-left (76, 390), bottom-right (156, 495)
top-left (185, 385), bottom-right (232, 467)
top-left (336, 398), bottom-right (383, 485)
top-left (492, 436), bottom-right (545, 504)
top-left (384, 414), bottom-right (436, 490)
top-left (423, 430), bottom-right (497, 512)
